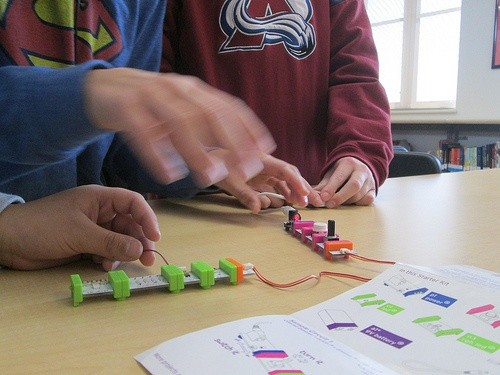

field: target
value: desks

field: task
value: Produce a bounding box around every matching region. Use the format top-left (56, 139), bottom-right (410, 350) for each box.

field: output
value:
top-left (0, 169), bottom-right (500, 375)
top-left (391, 117), bottom-right (500, 130)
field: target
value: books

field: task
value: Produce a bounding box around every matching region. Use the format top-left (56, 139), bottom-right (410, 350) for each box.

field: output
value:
top-left (438, 141), bottom-right (500, 173)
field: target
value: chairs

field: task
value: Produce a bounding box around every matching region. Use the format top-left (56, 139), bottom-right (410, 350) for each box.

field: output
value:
top-left (389, 152), bottom-right (440, 177)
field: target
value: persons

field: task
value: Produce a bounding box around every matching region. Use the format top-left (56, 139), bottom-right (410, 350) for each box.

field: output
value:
top-left (0, 0), bottom-right (309, 209)
top-left (0, 184), bottom-right (160, 272)
top-left (160, 0), bottom-right (395, 207)
